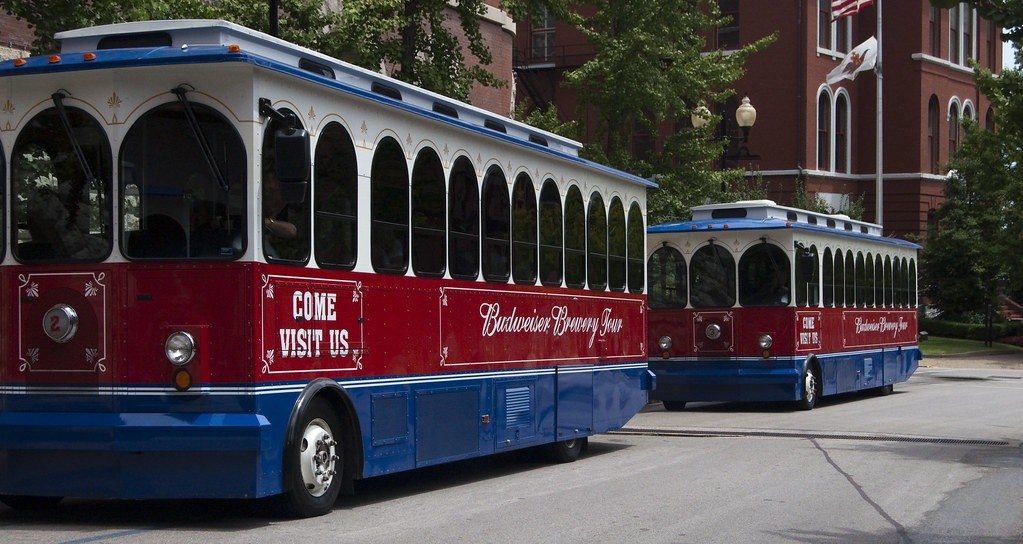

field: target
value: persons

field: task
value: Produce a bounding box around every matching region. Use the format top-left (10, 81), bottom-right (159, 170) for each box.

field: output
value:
top-left (217, 162), bottom-right (297, 259)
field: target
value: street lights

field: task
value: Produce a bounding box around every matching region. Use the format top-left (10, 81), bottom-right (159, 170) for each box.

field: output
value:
top-left (692, 95), bottom-right (762, 194)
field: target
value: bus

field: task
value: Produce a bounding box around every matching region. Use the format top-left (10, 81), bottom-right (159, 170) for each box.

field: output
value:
top-left (647, 201), bottom-right (921, 413)
top-left (0, 20), bottom-right (659, 523)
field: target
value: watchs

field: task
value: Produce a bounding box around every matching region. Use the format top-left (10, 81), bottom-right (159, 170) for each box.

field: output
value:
top-left (265, 216), bottom-right (274, 227)
